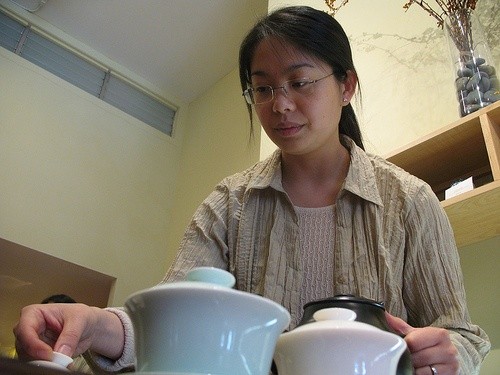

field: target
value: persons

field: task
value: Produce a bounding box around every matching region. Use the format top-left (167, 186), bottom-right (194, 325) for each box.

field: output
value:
top-left (10, 293), bottom-right (98, 375)
top-left (13, 6), bottom-right (491, 375)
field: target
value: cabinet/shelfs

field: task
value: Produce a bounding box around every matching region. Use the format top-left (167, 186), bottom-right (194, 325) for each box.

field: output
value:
top-left (389, 99), bottom-right (500, 247)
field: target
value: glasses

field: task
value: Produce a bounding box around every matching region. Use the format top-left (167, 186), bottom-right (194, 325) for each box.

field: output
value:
top-left (243, 70), bottom-right (338, 105)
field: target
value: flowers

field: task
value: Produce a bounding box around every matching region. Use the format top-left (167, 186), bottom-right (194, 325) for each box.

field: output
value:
top-left (325, 0), bottom-right (477, 61)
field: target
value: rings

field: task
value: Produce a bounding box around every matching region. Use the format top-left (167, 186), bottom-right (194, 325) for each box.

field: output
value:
top-left (429, 364), bottom-right (438, 375)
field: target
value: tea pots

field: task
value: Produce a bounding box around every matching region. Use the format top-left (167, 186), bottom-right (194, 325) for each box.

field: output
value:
top-left (270, 294), bottom-right (407, 375)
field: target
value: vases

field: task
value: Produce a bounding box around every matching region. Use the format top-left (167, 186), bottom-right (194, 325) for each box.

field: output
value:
top-left (444, 8), bottom-right (500, 118)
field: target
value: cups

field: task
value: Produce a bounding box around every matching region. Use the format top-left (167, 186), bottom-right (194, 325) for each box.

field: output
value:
top-left (274, 308), bottom-right (408, 375)
top-left (124, 267), bottom-right (292, 375)
